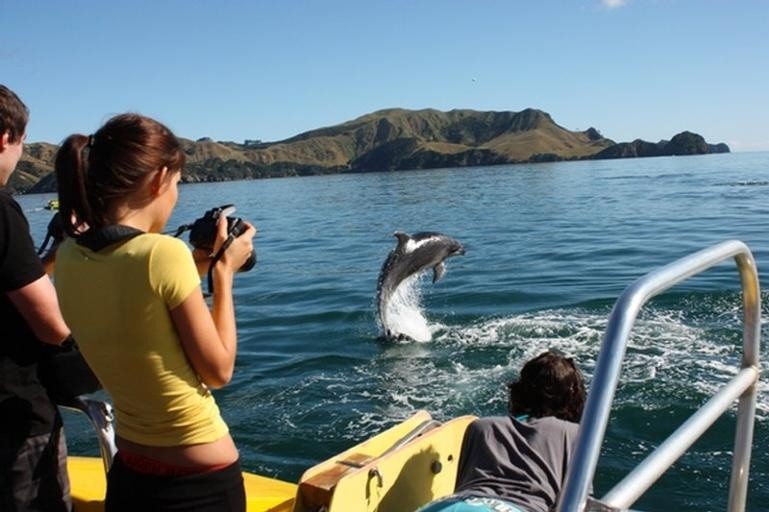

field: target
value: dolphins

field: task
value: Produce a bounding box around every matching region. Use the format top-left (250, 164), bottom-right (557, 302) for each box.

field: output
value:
top-left (376, 226), bottom-right (466, 343)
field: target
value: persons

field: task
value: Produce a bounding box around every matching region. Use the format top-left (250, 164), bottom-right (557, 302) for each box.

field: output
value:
top-left (0, 85), bottom-right (89, 512)
top-left (414, 350), bottom-right (594, 512)
top-left (55, 112), bottom-right (257, 511)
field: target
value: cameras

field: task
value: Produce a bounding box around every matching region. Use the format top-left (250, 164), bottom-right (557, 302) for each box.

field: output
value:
top-left (188, 204), bottom-right (257, 272)
